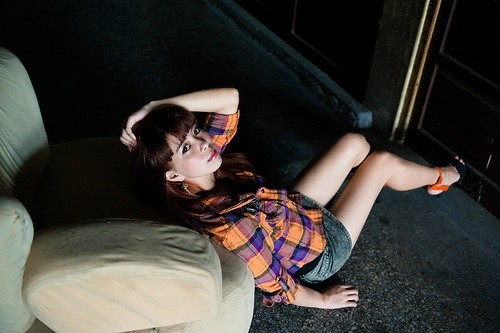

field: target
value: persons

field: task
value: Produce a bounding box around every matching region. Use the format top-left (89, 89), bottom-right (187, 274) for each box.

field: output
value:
top-left (120, 88), bottom-right (468, 310)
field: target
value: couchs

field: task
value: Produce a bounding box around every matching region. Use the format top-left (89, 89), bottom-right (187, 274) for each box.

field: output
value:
top-left (0, 47), bottom-right (257, 333)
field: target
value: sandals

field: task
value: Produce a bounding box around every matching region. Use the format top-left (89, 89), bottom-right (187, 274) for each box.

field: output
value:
top-left (427, 153), bottom-right (468, 198)
